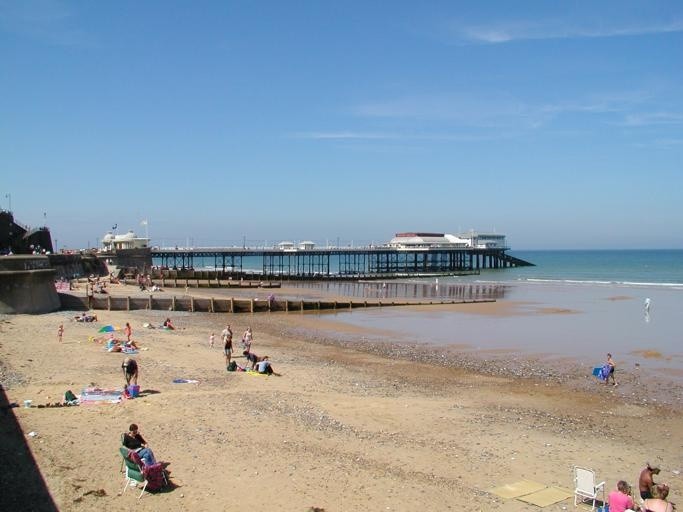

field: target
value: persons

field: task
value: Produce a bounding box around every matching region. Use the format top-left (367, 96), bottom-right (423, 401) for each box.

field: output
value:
top-left (124, 323), bottom-right (131, 340)
top-left (121, 424), bottom-right (156, 467)
top-left (640, 483), bottom-right (673, 512)
top-left (607, 480), bottom-right (640, 512)
top-left (241, 327), bottom-right (253, 351)
top-left (120, 358), bottom-right (137, 387)
top-left (165, 319), bottom-right (175, 330)
top-left (631, 361), bottom-right (640, 386)
top-left (623, 484), bottom-right (634, 505)
top-left (602, 353), bottom-right (618, 387)
top-left (220, 324), bottom-right (233, 347)
top-left (0, 237), bottom-right (195, 293)
top-left (642, 296), bottom-right (650, 312)
top-left (223, 334), bottom-right (233, 364)
top-left (258, 356), bottom-right (278, 374)
top-left (638, 460), bottom-right (677, 509)
top-left (56, 324), bottom-right (63, 341)
top-left (163, 317), bottom-right (169, 330)
top-left (241, 350), bottom-right (260, 371)
top-left (208, 332), bottom-right (215, 348)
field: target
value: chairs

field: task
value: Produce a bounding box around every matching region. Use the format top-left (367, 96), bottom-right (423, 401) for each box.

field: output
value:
top-left (119, 432), bottom-right (170, 500)
top-left (573, 466), bottom-right (606, 512)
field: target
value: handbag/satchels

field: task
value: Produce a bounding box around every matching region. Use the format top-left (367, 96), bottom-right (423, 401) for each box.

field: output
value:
top-left (227, 360), bottom-right (237, 371)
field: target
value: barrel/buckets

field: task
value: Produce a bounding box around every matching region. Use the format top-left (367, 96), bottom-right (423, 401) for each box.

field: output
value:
top-left (23, 400), bottom-right (33, 407)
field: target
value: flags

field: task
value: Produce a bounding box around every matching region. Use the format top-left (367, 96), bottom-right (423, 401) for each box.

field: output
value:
top-left (111, 224), bottom-right (117, 230)
